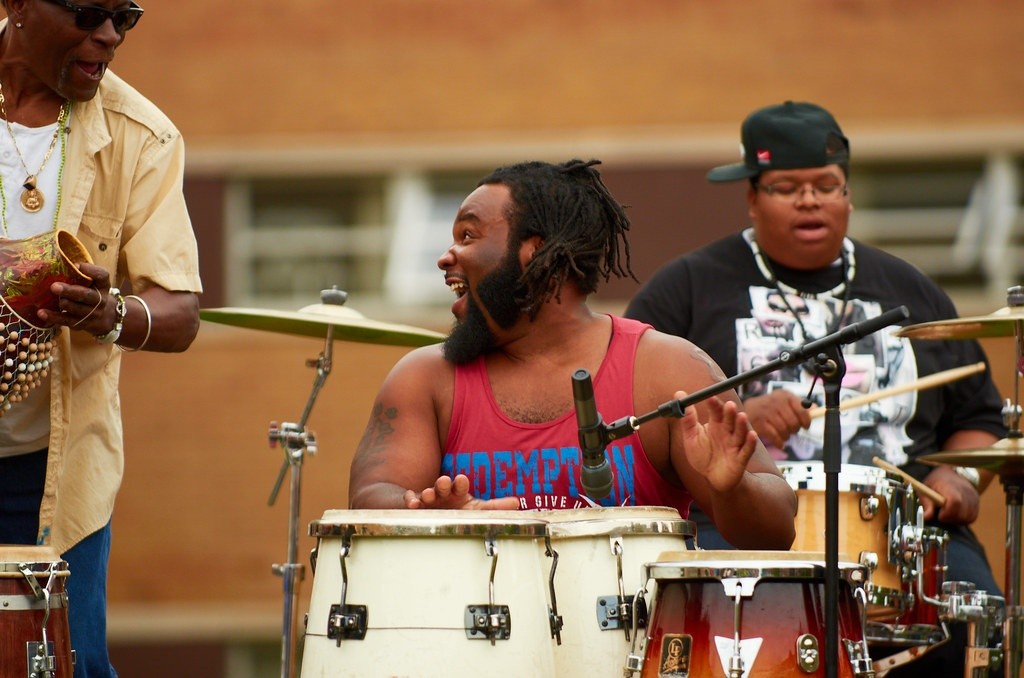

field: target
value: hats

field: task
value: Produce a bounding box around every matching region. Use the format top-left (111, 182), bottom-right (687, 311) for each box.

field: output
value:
top-left (705, 100), bottom-right (850, 182)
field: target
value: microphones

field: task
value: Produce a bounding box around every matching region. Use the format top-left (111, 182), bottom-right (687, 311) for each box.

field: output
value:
top-left (570, 368), bottom-right (614, 497)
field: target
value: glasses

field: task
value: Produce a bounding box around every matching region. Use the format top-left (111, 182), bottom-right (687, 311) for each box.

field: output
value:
top-left (46, 0), bottom-right (145, 33)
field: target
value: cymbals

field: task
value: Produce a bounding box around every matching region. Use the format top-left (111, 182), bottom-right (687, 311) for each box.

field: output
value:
top-left (916, 437), bottom-right (1024, 472)
top-left (890, 306), bottom-right (1024, 340)
top-left (199, 302), bottom-right (448, 347)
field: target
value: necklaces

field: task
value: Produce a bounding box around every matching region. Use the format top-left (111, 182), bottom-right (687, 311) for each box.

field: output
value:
top-left (0, 99), bottom-right (70, 237)
top-left (751, 238), bottom-right (855, 297)
top-left (0, 83), bottom-right (64, 212)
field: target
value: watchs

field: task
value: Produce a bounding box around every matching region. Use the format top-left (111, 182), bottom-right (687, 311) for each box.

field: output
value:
top-left (953, 466), bottom-right (980, 487)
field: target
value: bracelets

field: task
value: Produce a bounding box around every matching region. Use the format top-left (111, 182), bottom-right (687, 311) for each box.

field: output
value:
top-left (117, 295), bottom-right (151, 352)
top-left (94, 288), bottom-right (127, 344)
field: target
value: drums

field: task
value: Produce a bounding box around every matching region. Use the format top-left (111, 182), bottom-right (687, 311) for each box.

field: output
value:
top-left (864, 527), bottom-right (950, 644)
top-left (300, 509), bottom-right (556, 678)
top-left (774, 459), bottom-right (918, 622)
top-left (639, 549), bottom-right (870, 678)
top-left (0, 544), bottom-right (74, 678)
top-left (544, 506), bottom-right (697, 678)
top-left (865, 594), bottom-right (1005, 678)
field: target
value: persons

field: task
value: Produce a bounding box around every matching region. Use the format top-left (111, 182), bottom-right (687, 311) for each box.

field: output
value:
top-left (624, 102), bottom-right (1009, 596)
top-left (349, 160), bottom-right (797, 550)
top-left (0, 0), bottom-right (203, 678)
